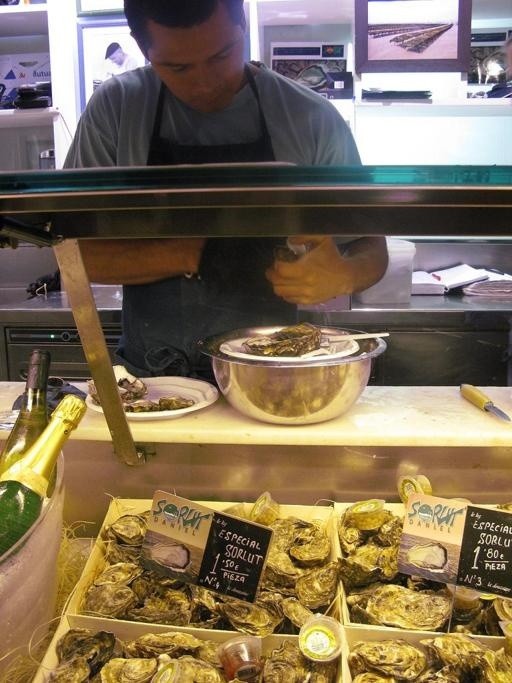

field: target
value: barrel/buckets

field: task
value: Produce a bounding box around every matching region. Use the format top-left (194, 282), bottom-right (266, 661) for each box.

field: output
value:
top-left (2, 111), bottom-right (53, 170)
top-left (0, 426), bottom-right (65, 681)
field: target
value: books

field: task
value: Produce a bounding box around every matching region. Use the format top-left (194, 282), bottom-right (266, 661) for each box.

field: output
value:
top-left (460, 267), bottom-right (512, 298)
top-left (461, 294), bottom-right (512, 306)
top-left (412, 294), bottom-right (486, 310)
top-left (411, 262), bottom-right (491, 296)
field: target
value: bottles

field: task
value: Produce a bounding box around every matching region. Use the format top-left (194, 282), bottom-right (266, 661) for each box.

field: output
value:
top-left (0, 345), bottom-right (87, 558)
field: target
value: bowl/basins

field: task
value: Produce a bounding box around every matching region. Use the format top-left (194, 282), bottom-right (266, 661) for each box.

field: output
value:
top-left (195, 324), bottom-right (389, 426)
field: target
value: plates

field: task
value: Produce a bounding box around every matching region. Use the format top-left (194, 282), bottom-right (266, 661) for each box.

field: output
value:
top-left (85, 374), bottom-right (220, 423)
top-left (218, 335), bottom-right (360, 364)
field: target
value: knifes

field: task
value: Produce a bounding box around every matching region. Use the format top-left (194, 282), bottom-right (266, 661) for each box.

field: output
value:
top-left (458, 381), bottom-right (512, 427)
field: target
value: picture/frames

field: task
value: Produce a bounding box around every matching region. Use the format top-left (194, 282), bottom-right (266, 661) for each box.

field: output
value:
top-left (351, 0), bottom-right (473, 72)
top-left (73, 0), bottom-right (150, 117)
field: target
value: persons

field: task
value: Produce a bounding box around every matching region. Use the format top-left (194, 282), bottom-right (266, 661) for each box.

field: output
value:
top-left (105, 42), bottom-right (137, 82)
top-left (471, 34), bottom-right (511, 98)
top-left (60, 1), bottom-right (389, 386)
